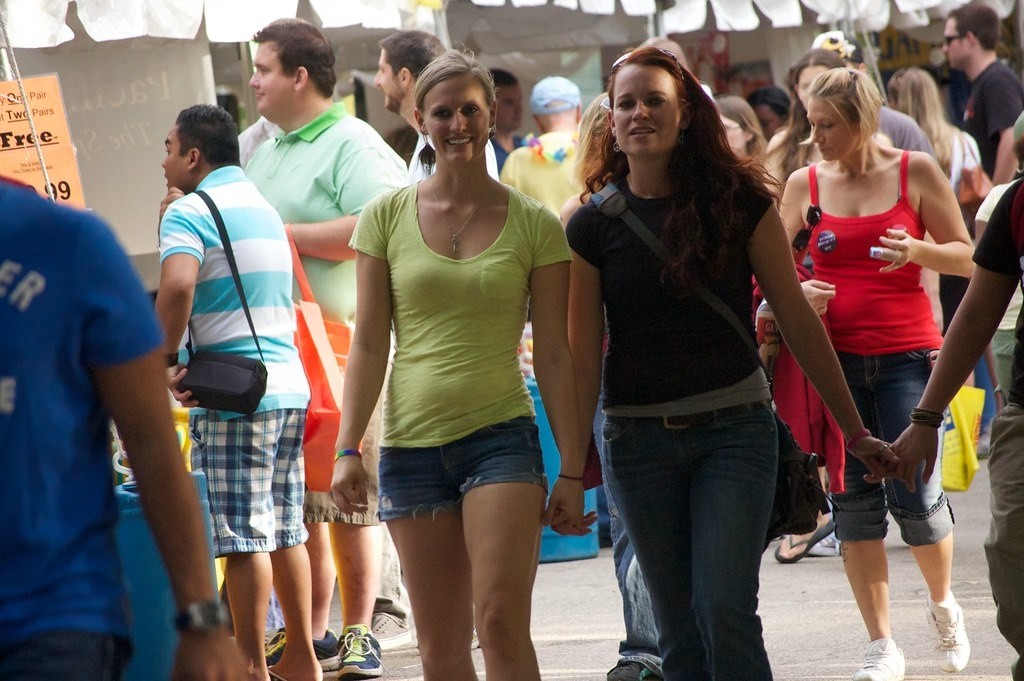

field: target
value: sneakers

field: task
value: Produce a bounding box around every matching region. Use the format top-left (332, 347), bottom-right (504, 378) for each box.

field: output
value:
top-left (926, 600), bottom-right (971, 673)
top-left (263, 628), bottom-right (341, 671)
top-left (854, 640), bottom-right (907, 681)
top-left (336, 625), bottom-right (395, 679)
top-left (607, 662), bottom-right (663, 681)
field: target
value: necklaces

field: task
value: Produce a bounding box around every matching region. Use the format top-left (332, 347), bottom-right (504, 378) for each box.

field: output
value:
top-left (429, 175), bottom-right (492, 252)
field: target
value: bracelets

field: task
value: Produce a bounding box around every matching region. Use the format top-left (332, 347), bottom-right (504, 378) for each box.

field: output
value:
top-left (335, 449), bottom-right (361, 462)
top-left (558, 474), bottom-right (583, 481)
top-left (910, 407), bottom-right (944, 429)
top-left (166, 350), bottom-right (178, 367)
top-left (846, 429), bottom-right (872, 451)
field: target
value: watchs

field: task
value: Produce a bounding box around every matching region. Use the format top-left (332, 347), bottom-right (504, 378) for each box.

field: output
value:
top-left (175, 598), bottom-right (228, 634)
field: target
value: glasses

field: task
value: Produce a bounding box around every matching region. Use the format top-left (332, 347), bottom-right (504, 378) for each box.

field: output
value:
top-left (944, 35), bottom-right (965, 46)
top-left (792, 204), bottom-right (823, 254)
top-left (894, 68), bottom-right (909, 84)
top-left (611, 47), bottom-right (685, 80)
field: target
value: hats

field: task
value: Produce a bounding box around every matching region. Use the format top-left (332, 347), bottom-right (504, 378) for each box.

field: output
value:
top-left (528, 76), bottom-right (578, 115)
top-left (812, 30), bottom-right (863, 63)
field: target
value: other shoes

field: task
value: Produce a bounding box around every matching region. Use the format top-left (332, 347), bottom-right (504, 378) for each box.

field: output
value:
top-left (371, 612), bottom-right (418, 651)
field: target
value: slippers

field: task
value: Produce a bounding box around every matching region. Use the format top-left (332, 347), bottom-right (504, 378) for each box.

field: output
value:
top-left (774, 519), bottom-right (836, 562)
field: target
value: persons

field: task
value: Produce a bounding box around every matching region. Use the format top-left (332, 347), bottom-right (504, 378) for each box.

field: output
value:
top-left (887, 176), bottom-right (1024, 681)
top-left (697, 4), bottom-right (1024, 563)
top-left (544, 46), bottom-right (897, 681)
top-left (244, 17), bottom-right (412, 681)
top-left (151, 104), bottom-right (323, 681)
top-left (0, 175), bottom-right (248, 681)
top-left (373, 30), bottom-right (668, 681)
top-left (330, 47), bottom-right (586, 681)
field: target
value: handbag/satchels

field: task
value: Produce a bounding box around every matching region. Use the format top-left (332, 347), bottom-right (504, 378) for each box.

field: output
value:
top-left (764, 410), bottom-right (830, 549)
top-left (941, 385), bottom-right (985, 490)
top-left (177, 351), bottom-right (266, 412)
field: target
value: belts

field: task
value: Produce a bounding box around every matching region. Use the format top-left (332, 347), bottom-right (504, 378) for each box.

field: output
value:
top-left (605, 399), bottom-right (771, 430)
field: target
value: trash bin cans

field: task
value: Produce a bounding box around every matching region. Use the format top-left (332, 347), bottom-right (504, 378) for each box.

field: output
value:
top-left (112, 472), bottom-right (220, 681)
top-left (525, 374), bottom-right (599, 562)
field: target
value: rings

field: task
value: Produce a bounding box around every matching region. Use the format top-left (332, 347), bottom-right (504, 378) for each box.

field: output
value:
top-left (879, 445), bottom-right (888, 452)
